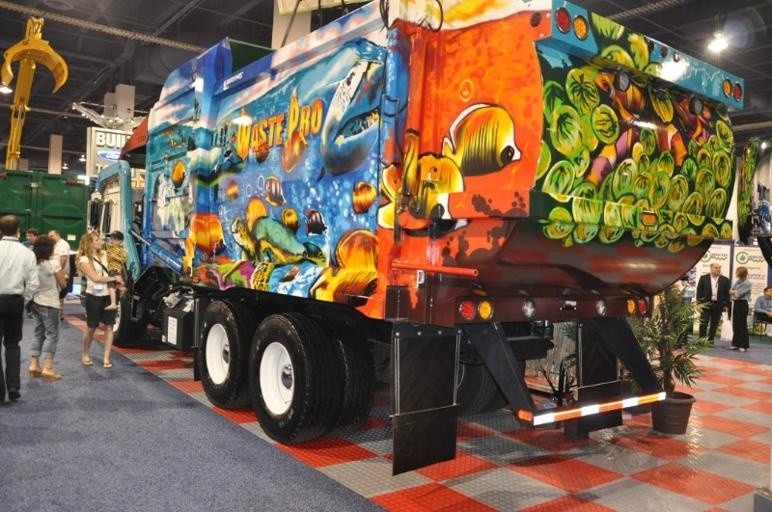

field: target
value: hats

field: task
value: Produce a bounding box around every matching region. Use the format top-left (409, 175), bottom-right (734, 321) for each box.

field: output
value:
top-left (105, 230), bottom-right (124, 241)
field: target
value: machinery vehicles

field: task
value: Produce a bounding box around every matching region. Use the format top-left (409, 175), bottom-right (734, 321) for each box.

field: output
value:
top-left (1, 18), bottom-right (90, 299)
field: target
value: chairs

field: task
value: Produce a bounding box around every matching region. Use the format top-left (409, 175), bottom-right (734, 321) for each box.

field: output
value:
top-left (751, 308), bottom-right (772, 343)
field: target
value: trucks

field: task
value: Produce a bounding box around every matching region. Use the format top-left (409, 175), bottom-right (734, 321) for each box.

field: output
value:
top-left (80, 1), bottom-right (746, 477)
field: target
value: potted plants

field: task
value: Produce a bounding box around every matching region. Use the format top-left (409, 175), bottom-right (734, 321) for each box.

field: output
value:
top-left (638, 283), bottom-right (714, 435)
top-left (621, 311), bottom-right (666, 416)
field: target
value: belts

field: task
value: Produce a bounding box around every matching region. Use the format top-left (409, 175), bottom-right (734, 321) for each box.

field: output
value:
top-left (711, 300), bottom-right (717, 303)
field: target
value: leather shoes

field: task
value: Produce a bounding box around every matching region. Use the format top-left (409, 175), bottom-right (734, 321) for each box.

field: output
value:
top-left (6, 386), bottom-right (22, 401)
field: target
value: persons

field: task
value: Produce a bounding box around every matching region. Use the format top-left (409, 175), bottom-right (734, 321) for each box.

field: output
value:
top-left (0, 214), bottom-right (73, 402)
top-left (754, 287), bottom-right (772, 325)
top-left (728, 267), bottom-right (751, 352)
top-left (103, 231), bottom-right (128, 311)
top-left (696, 263), bottom-right (730, 346)
top-left (76, 230), bottom-right (122, 369)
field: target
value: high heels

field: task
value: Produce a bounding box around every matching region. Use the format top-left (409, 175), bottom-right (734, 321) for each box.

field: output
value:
top-left (29, 366), bottom-right (43, 377)
top-left (42, 367), bottom-right (62, 382)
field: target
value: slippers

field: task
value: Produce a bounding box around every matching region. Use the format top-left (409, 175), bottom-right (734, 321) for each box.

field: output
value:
top-left (81, 357), bottom-right (92, 366)
top-left (101, 359), bottom-right (113, 368)
